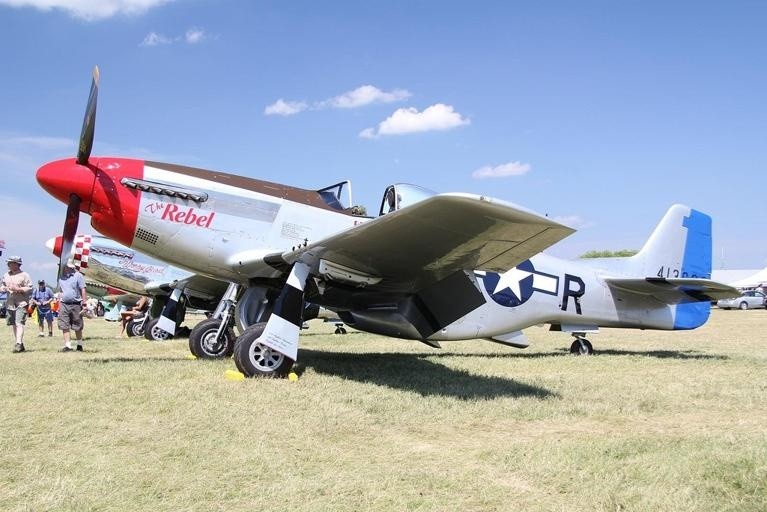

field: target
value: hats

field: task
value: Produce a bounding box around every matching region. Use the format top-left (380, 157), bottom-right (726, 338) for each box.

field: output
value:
top-left (38, 280), bottom-right (45, 284)
top-left (57, 258), bottom-right (76, 268)
top-left (6, 256), bottom-right (22, 264)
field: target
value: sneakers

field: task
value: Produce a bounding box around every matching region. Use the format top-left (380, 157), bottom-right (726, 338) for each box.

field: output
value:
top-left (76, 345), bottom-right (83, 352)
top-left (58, 346), bottom-right (73, 352)
top-left (13, 342), bottom-right (25, 353)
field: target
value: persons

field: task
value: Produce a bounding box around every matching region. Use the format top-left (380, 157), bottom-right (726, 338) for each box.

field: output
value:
top-left (0, 256), bottom-right (148, 353)
top-left (741, 284), bottom-right (764, 297)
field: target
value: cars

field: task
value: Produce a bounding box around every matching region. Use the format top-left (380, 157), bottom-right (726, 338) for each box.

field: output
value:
top-left (716, 289), bottom-right (767, 311)
